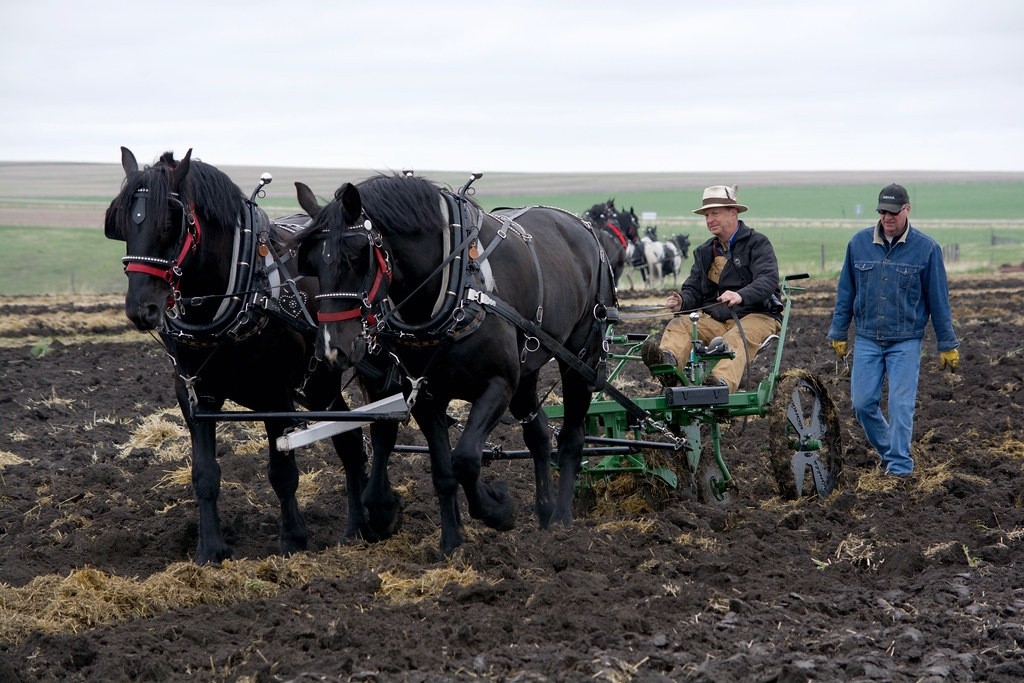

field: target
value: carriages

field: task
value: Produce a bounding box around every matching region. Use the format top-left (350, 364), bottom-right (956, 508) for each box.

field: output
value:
top-left (104, 146), bottom-right (839, 569)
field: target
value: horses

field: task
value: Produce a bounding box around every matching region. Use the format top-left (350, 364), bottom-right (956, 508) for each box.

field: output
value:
top-left (101, 143), bottom-right (691, 566)
top-left (615, 222), bottom-right (690, 291)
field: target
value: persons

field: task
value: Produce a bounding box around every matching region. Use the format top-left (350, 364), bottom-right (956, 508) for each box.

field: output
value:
top-left (826, 183), bottom-right (958, 475)
top-left (640, 184), bottom-right (782, 395)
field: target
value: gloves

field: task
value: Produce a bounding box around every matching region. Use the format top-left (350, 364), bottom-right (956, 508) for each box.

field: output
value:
top-left (832, 339), bottom-right (847, 361)
top-left (939, 348), bottom-right (959, 374)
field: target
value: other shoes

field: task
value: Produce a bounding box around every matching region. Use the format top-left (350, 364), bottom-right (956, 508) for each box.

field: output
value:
top-left (705, 373), bottom-right (727, 388)
top-left (876, 459), bottom-right (890, 477)
top-left (641, 340), bottom-right (679, 388)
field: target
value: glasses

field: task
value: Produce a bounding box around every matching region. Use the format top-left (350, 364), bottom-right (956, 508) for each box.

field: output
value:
top-left (878, 207), bottom-right (906, 216)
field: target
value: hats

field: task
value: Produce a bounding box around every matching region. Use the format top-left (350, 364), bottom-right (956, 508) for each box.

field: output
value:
top-left (875, 182), bottom-right (910, 214)
top-left (691, 185), bottom-right (749, 216)
